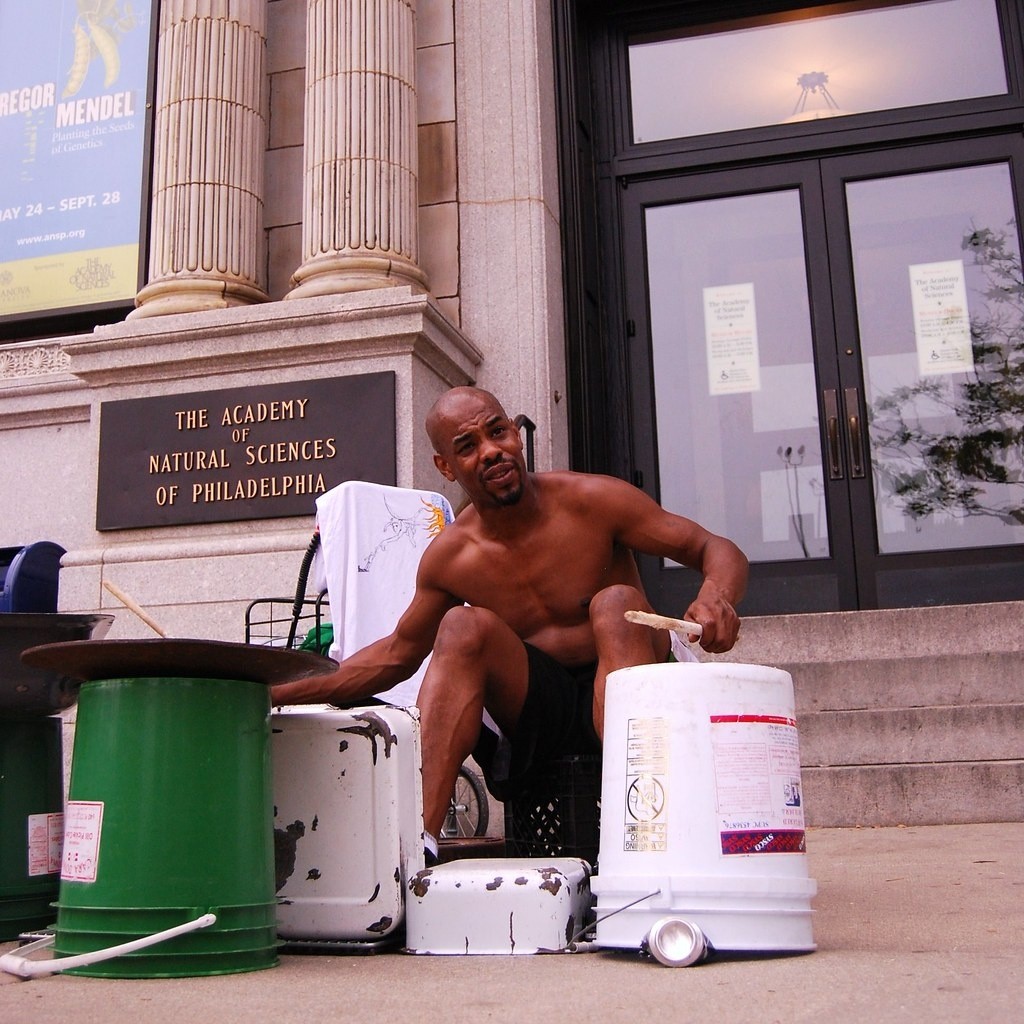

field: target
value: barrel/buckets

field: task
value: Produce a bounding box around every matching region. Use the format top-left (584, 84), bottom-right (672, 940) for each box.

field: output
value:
top-left (1, 680), bottom-right (286, 979)
top-left (0, 708), bottom-right (65, 944)
top-left (560, 653), bottom-right (823, 960)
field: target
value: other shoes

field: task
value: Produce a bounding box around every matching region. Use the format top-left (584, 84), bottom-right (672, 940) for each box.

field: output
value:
top-left (425, 846), bottom-right (439, 868)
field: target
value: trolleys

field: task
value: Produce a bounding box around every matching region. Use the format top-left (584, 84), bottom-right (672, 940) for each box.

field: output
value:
top-left (243, 480), bottom-right (490, 839)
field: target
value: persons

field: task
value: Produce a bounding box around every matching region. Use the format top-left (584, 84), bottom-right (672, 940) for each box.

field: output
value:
top-left (270, 385), bottom-right (750, 870)
